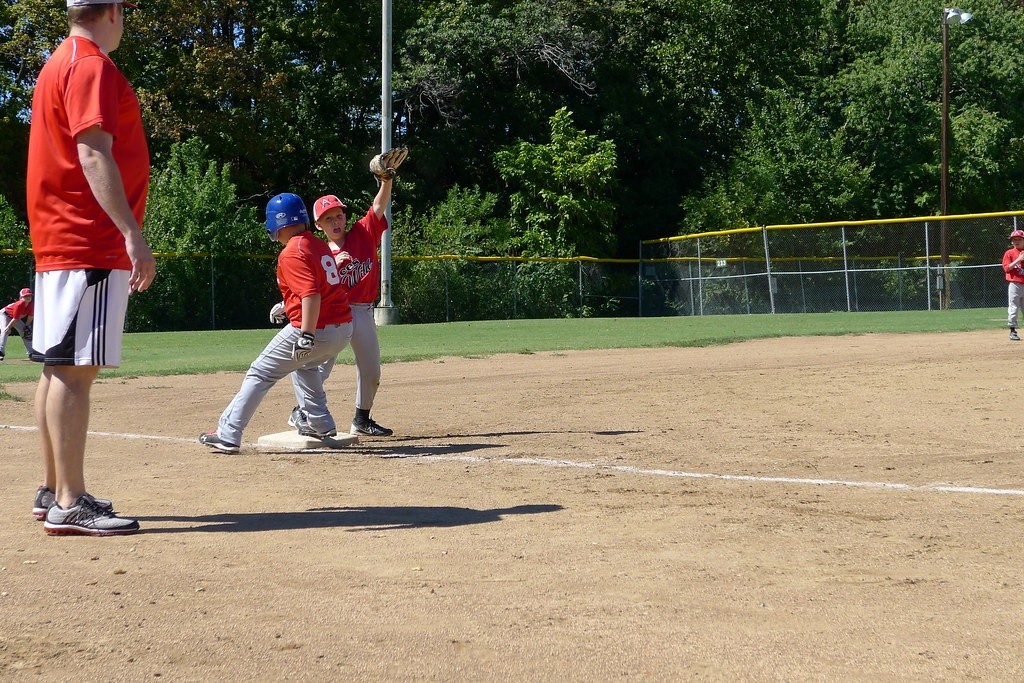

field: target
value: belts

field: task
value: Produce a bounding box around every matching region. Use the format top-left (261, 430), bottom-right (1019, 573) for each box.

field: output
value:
top-left (4, 311), bottom-right (13, 319)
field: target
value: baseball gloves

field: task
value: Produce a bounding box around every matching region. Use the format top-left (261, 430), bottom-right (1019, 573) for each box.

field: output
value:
top-left (369, 145), bottom-right (407, 181)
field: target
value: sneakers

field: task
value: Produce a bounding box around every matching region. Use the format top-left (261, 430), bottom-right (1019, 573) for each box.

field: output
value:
top-left (32, 485), bottom-right (112, 519)
top-left (350, 418), bottom-right (393, 436)
top-left (299, 426), bottom-right (337, 438)
top-left (43, 495), bottom-right (140, 535)
top-left (1009, 331), bottom-right (1020, 340)
top-left (288, 407), bottom-right (313, 433)
top-left (199, 430), bottom-right (239, 454)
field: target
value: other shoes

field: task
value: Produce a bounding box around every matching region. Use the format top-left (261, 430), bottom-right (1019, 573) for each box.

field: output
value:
top-left (0, 355), bottom-right (4, 360)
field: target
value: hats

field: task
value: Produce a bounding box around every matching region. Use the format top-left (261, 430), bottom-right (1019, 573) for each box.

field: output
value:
top-left (313, 195), bottom-right (348, 221)
top-left (19, 288), bottom-right (32, 296)
top-left (67, 0), bottom-right (138, 8)
top-left (1009, 230), bottom-right (1024, 239)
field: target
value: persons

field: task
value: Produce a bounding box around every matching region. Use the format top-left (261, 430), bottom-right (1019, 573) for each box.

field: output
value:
top-left (287, 144), bottom-right (394, 438)
top-left (0, 288), bottom-right (34, 360)
top-left (199, 193), bottom-right (353, 456)
top-left (1002, 230), bottom-right (1024, 340)
top-left (26, 1), bottom-right (159, 538)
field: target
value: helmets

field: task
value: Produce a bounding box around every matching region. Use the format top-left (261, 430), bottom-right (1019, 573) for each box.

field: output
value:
top-left (265, 193), bottom-right (310, 241)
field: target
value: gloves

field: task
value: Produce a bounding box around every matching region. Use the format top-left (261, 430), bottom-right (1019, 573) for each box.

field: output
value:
top-left (269, 301), bottom-right (285, 324)
top-left (291, 332), bottom-right (315, 361)
